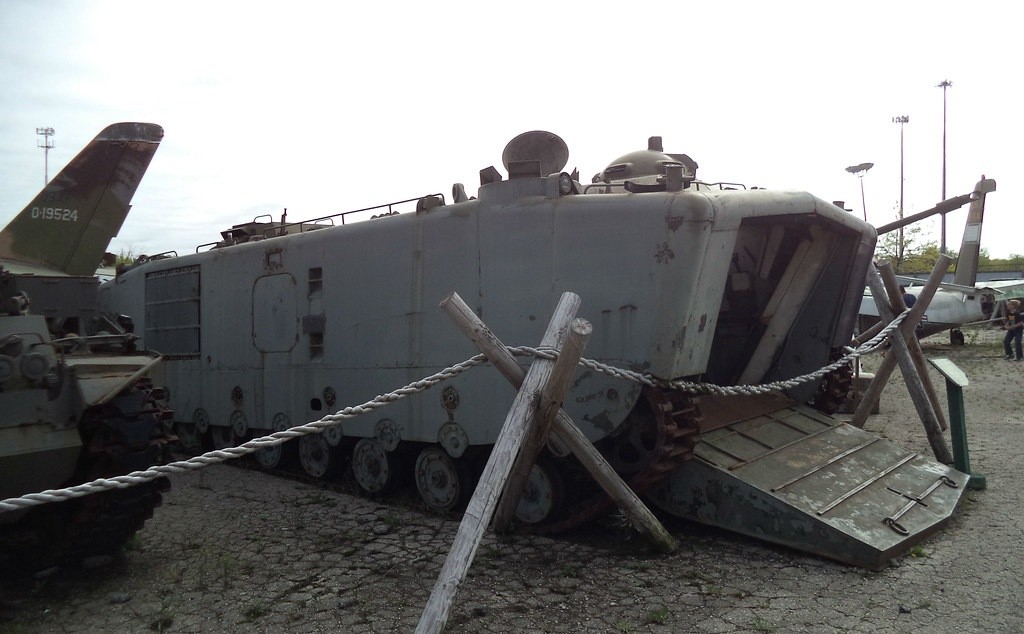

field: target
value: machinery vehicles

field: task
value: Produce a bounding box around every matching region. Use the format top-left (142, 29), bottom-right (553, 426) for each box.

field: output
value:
top-left (0, 263), bottom-right (178, 582)
top-left (0, 123), bottom-right (973, 573)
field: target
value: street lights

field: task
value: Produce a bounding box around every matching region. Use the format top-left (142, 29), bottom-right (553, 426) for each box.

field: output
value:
top-left (891, 115), bottom-right (910, 258)
top-left (935, 80), bottom-right (954, 253)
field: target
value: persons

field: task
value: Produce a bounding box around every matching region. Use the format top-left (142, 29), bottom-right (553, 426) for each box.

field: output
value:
top-left (1002, 300), bottom-right (1024, 362)
top-left (899, 285), bottom-right (924, 343)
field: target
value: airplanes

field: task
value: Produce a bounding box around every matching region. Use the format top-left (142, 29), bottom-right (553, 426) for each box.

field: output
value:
top-left (854, 175), bottom-right (1005, 347)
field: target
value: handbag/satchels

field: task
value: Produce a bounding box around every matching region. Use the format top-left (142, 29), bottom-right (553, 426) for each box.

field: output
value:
top-left (950, 327), bottom-right (964, 346)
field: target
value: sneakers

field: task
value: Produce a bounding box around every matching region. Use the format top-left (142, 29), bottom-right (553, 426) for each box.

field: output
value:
top-left (1013, 356), bottom-right (1023, 360)
top-left (1004, 354), bottom-right (1014, 360)
